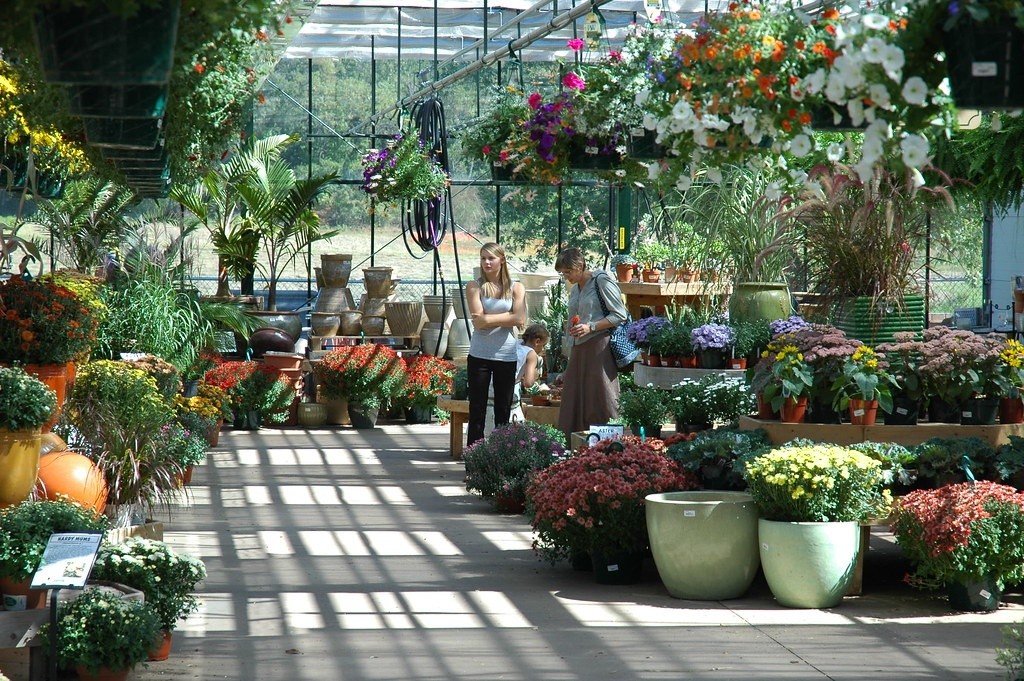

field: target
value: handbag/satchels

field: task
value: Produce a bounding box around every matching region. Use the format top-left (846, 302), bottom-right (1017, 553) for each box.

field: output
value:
top-left (610, 315), bottom-right (641, 368)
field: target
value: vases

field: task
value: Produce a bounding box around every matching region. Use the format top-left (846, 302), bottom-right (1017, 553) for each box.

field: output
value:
top-left (0, 428), bottom-right (42, 511)
top-left (7, 362), bottom-right (66, 434)
top-left (76, 664), bottom-right (129, 681)
top-left (105, 501), bottom-right (147, 528)
top-left (0, 0), bottom-right (184, 197)
top-left (0, 576), bottom-right (47, 610)
top-left (488, 0), bottom-right (1024, 609)
top-left (66, 361), bottom-right (77, 389)
top-left (164, 253), bottom-right (474, 489)
top-left (148, 629), bottom-right (172, 662)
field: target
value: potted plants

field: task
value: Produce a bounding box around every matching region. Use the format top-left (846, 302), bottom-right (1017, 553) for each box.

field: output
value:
top-left (215, 134), bottom-right (344, 340)
top-left (168, 173), bottom-right (265, 313)
top-left (531, 281), bottom-right (568, 384)
top-left (755, 165), bottom-right (978, 341)
top-left (650, 159), bottom-right (792, 323)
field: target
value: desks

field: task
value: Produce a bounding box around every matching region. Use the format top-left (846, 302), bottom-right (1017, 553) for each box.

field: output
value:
top-left (437, 396), bottom-right (561, 459)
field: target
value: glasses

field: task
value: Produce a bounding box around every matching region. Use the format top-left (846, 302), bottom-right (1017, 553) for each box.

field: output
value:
top-left (559, 270), bottom-right (574, 276)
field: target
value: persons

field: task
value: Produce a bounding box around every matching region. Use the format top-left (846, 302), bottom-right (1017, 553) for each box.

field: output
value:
top-left (555, 247), bottom-right (628, 449)
top-left (467, 242), bottom-right (527, 447)
top-left (485, 323), bottom-right (549, 441)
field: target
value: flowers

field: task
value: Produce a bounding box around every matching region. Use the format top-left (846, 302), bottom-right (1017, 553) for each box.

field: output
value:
top-left (0, 0), bottom-right (296, 184)
top-left (314, 312), bottom-right (1024, 681)
top-left (0, 271), bottom-right (299, 681)
top-left (359, 0), bottom-right (1024, 210)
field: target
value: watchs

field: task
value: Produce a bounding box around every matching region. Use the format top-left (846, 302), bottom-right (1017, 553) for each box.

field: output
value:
top-left (588, 322), bottom-right (596, 334)
